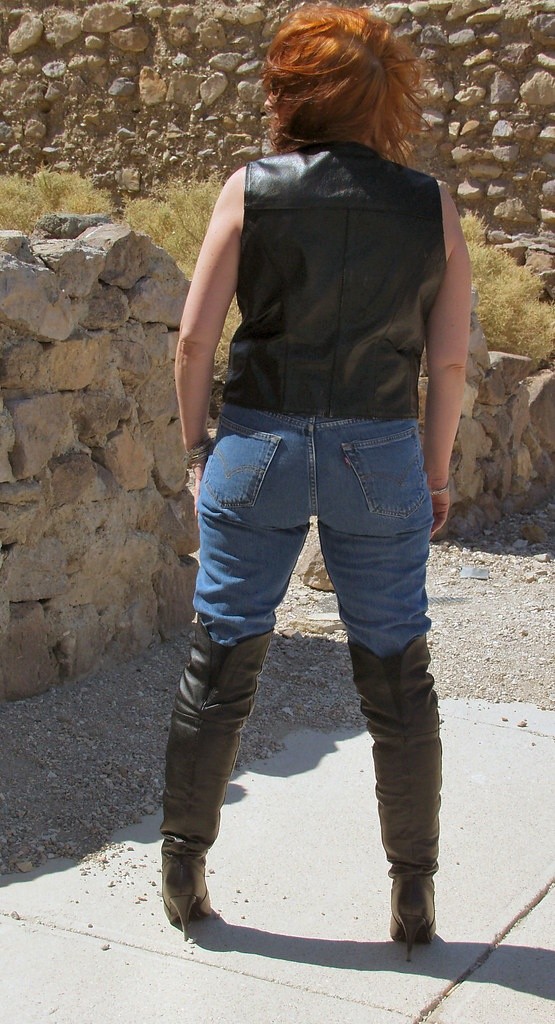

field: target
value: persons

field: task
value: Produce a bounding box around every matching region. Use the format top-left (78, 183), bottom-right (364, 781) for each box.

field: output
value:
top-left (161, 6), bottom-right (471, 962)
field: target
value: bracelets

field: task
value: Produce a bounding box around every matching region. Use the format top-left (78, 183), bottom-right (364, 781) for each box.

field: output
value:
top-left (185, 434), bottom-right (213, 468)
top-left (431, 482), bottom-right (449, 496)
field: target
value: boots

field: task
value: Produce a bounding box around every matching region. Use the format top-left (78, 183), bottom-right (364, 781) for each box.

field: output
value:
top-left (162, 615), bottom-right (274, 941)
top-left (350, 633), bottom-right (441, 963)
